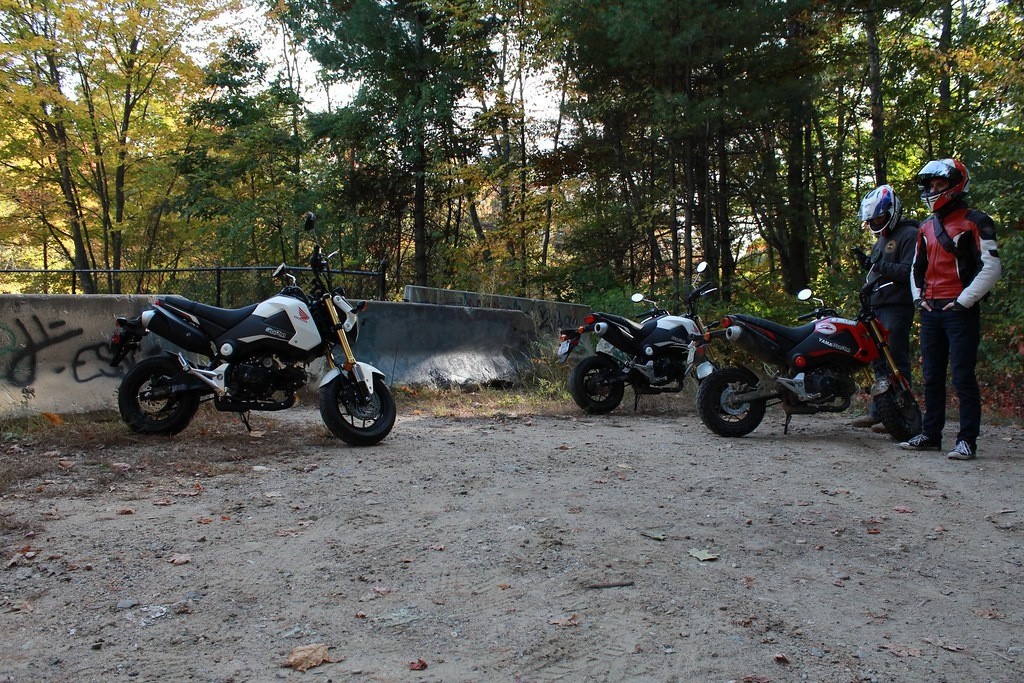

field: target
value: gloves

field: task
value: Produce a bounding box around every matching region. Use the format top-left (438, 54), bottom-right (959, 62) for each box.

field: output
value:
top-left (864, 258), bottom-right (872, 268)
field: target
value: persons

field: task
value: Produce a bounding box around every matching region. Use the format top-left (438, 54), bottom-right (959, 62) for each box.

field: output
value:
top-left (900, 157), bottom-right (1003, 460)
top-left (851, 183), bottom-right (921, 433)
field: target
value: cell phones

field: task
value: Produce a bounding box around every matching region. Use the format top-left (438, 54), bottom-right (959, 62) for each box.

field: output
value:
top-left (851, 247), bottom-right (866, 256)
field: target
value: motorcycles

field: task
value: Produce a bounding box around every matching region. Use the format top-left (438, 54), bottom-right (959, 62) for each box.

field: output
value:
top-left (692, 248), bottom-right (924, 443)
top-left (107, 209), bottom-right (397, 448)
top-left (554, 260), bottom-right (722, 417)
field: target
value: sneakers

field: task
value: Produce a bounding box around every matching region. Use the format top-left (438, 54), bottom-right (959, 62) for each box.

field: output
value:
top-left (946, 440), bottom-right (977, 459)
top-left (898, 433), bottom-right (942, 451)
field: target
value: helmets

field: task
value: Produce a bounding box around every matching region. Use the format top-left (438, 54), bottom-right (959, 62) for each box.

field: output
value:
top-left (857, 184), bottom-right (903, 237)
top-left (915, 158), bottom-right (970, 213)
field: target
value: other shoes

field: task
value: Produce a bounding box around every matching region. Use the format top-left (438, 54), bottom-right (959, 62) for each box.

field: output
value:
top-left (850, 412), bottom-right (882, 426)
top-left (871, 422), bottom-right (889, 433)
